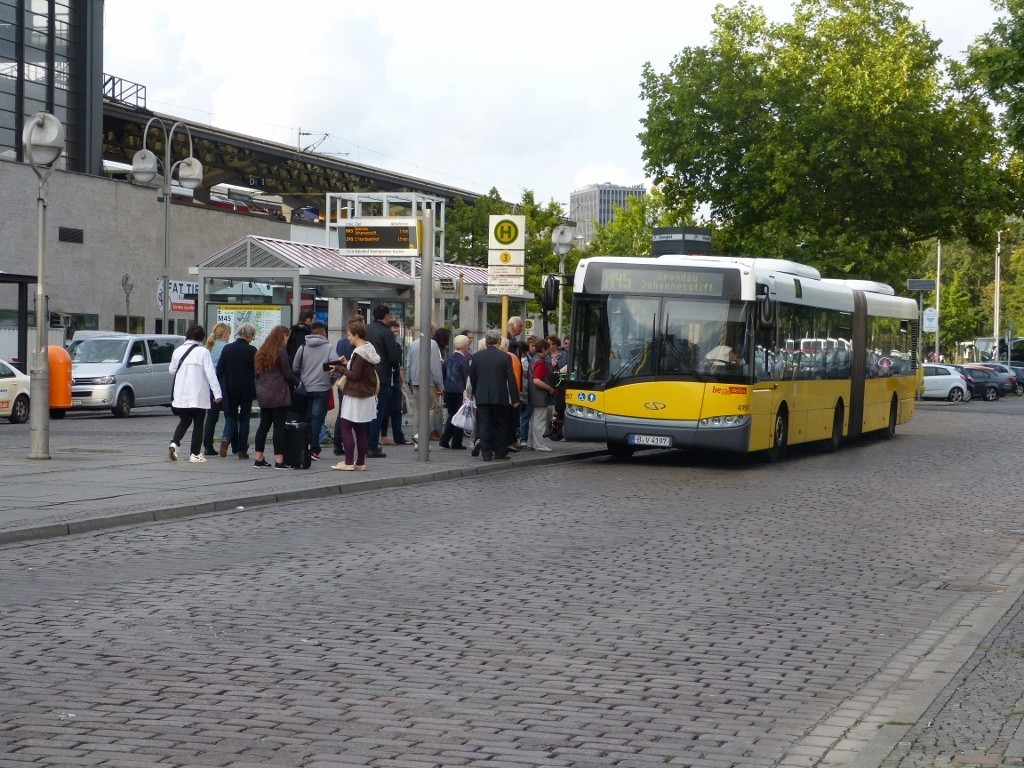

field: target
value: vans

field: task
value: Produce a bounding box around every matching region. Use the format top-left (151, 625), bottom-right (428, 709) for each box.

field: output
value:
top-left (66, 333), bottom-right (186, 418)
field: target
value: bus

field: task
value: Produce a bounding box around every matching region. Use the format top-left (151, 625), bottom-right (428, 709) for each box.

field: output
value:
top-left (964, 337), bottom-right (1024, 362)
top-left (563, 253), bottom-right (920, 463)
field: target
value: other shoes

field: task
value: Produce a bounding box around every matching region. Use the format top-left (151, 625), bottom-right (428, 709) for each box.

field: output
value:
top-left (311, 451), bottom-right (321, 460)
top-left (220, 439), bottom-right (229, 458)
top-left (205, 447), bottom-right (218, 455)
top-left (472, 439), bottom-right (552, 461)
top-left (333, 449), bottom-right (345, 455)
top-left (366, 447), bottom-right (386, 458)
top-left (332, 461), bottom-right (365, 471)
top-left (238, 452), bottom-right (249, 459)
top-left (379, 431), bottom-right (467, 451)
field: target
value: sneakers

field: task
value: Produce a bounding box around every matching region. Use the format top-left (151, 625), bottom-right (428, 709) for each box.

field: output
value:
top-left (169, 443), bottom-right (179, 461)
top-left (189, 453), bottom-right (207, 462)
top-left (274, 462), bottom-right (294, 470)
top-left (254, 458), bottom-right (272, 469)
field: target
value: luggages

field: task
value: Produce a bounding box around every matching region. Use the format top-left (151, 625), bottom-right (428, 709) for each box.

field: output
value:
top-left (284, 420), bottom-right (312, 469)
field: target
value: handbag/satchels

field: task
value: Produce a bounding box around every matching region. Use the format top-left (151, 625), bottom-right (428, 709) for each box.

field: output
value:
top-left (171, 398), bottom-right (178, 415)
top-left (328, 388), bottom-right (334, 410)
top-left (401, 388), bottom-right (410, 415)
top-left (293, 382), bottom-right (307, 395)
top-left (451, 398), bottom-right (477, 431)
top-left (337, 375), bottom-right (346, 391)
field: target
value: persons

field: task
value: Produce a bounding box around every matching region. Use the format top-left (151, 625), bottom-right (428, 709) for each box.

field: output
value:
top-left (168, 306), bottom-right (588, 471)
top-left (704, 345), bottom-right (746, 366)
top-left (930, 353), bottom-right (945, 363)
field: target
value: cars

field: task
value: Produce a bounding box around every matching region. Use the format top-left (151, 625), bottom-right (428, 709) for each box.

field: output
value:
top-left (754, 337), bottom-right (912, 381)
top-left (0, 359), bottom-right (32, 425)
top-left (946, 364), bottom-right (975, 402)
top-left (963, 361), bottom-right (1024, 401)
top-left (921, 363), bottom-right (967, 403)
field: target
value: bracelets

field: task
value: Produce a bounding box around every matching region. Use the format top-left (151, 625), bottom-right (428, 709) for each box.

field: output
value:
top-left (548, 386), bottom-right (552, 392)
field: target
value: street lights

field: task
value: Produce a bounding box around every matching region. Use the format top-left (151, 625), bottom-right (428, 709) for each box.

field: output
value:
top-left (22, 112), bottom-right (67, 459)
top-left (121, 273), bottom-right (134, 334)
top-left (552, 225), bottom-right (573, 341)
top-left (132, 117), bottom-right (204, 333)
